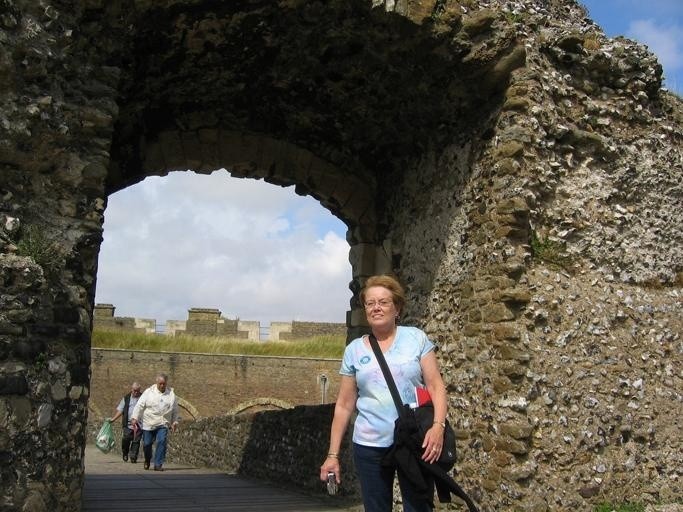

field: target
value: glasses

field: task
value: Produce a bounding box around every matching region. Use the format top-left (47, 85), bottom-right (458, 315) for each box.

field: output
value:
top-left (364, 300), bottom-right (394, 308)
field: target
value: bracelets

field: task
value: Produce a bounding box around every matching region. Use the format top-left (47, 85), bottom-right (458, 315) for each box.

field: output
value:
top-left (326, 452), bottom-right (339, 459)
top-left (430, 420), bottom-right (447, 428)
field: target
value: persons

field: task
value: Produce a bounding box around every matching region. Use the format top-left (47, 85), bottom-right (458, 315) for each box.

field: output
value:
top-left (130, 372), bottom-right (181, 471)
top-left (108, 382), bottom-right (145, 463)
top-left (320, 274), bottom-right (448, 511)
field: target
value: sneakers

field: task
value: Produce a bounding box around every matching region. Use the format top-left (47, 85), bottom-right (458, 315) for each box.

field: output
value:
top-left (144, 463), bottom-right (164, 471)
top-left (123, 454), bottom-right (136, 463)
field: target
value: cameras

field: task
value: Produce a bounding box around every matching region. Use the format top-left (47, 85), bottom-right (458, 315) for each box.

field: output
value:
top-left (326, 472), bottom-right (339, 496)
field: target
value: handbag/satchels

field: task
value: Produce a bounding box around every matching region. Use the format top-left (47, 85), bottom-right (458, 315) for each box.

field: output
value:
top-left (96, 418), bottom-right (116, 455)
top-left (394, 403), bottom-right (458, 484)
